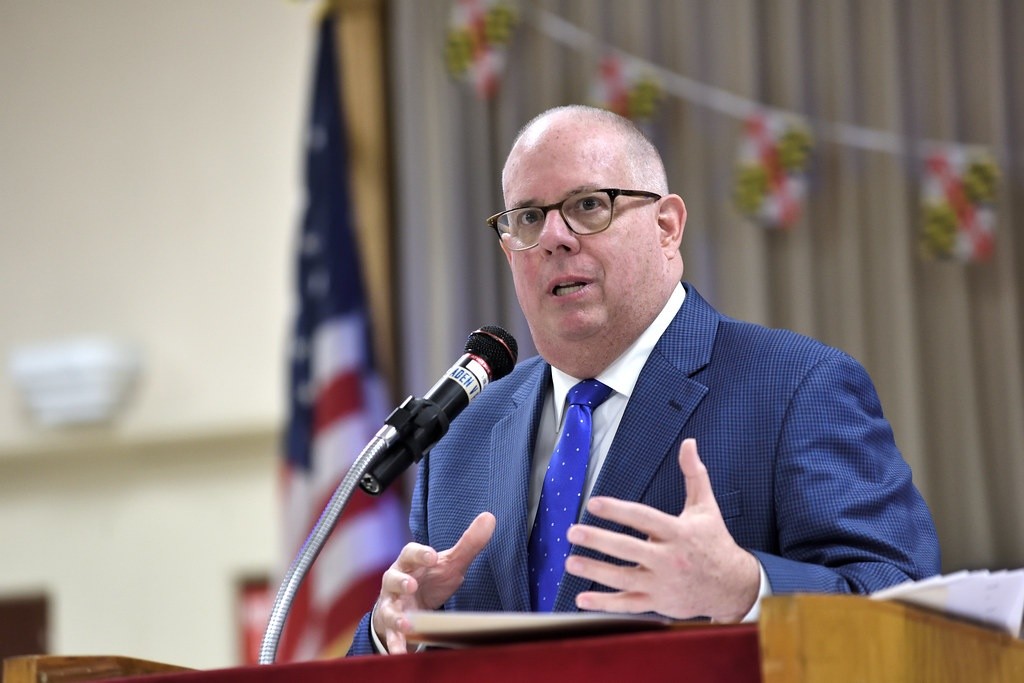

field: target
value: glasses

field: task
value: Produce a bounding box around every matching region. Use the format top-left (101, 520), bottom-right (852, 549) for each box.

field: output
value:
top-left (487, 189), bottom-right (661, 251)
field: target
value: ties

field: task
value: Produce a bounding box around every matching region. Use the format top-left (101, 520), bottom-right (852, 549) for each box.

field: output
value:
top-left (528, 379), bottom-right (611, 611)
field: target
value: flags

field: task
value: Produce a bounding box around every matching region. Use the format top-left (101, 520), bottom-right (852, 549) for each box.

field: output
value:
top-left (434, 0), bottom-right (525, 100)
top-left (731, 110), bottom-right (813, 230)
top-left (275, 8), bottom-right (410, 658)
top-left (575, 54), bottom-right (680, 152)
top-left (915, 139), bottom-right (1002, 268)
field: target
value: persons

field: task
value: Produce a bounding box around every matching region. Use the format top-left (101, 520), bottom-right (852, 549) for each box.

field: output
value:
top-left (344, 101), bottom-right (939, 661)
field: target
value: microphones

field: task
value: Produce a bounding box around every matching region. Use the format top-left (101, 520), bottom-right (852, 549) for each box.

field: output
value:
top-left (357, 324), bottom-right (518, 494)
